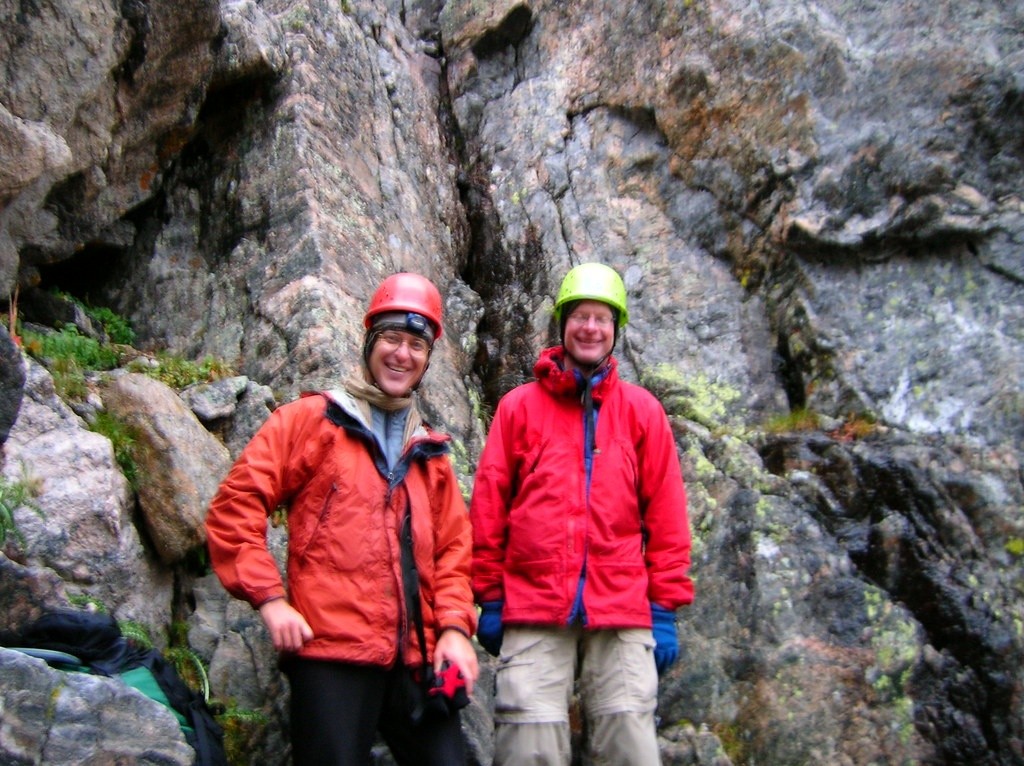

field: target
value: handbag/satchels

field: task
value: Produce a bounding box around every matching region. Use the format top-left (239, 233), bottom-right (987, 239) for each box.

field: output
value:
top-left (0, 599), bottom-right (227, 766)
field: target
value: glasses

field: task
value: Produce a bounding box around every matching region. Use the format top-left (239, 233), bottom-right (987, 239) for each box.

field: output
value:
top-left (376, 332), bottom-right (432, 355)
top-left (567, 311), bottom-right (616, 325)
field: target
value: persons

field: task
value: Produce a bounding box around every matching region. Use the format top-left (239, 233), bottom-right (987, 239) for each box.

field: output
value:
top-left (204, 272), bottom-right (478, 766)
top-left (470, 262), bottom-right (695, 766)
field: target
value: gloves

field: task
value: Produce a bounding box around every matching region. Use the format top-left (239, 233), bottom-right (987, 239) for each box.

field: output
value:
top-left (476, 600), bottom-right (506, 657)
top-left (650, 607), bottom-right (679, 668)
top-left (413, 661), bottom-right (473, 713)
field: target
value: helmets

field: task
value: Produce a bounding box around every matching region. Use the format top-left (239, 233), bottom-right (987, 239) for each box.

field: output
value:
top-left (554, 262), bottom-right (630, 337)
top-left (364, 272), bottom-right (442, 347)
